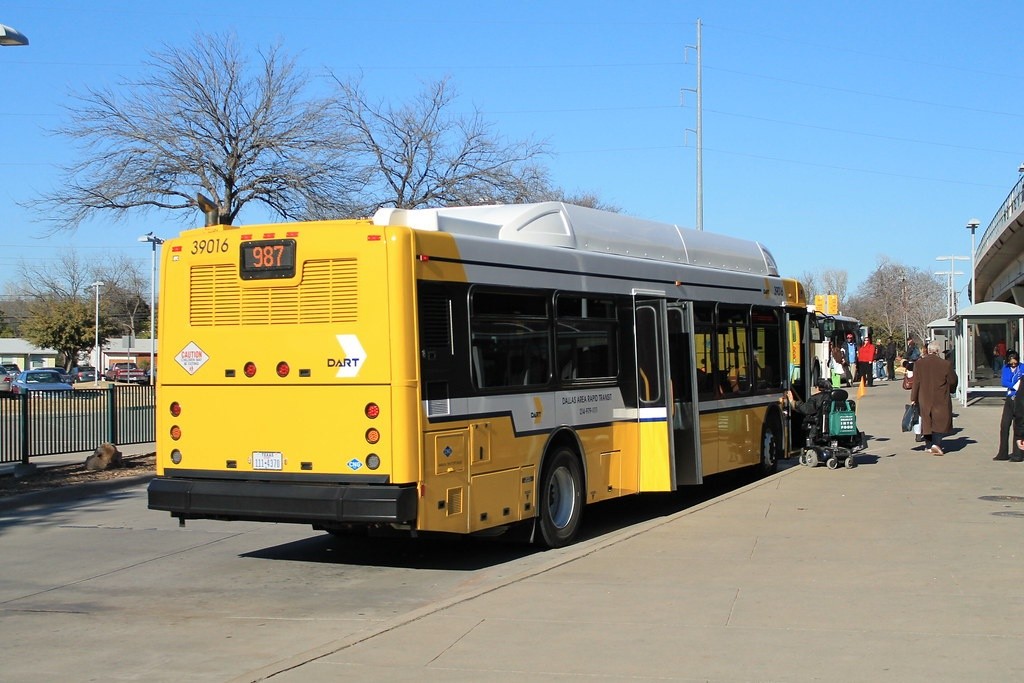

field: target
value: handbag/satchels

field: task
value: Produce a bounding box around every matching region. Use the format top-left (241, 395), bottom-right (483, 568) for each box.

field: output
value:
top-left (850, 428), bottom-right (867, 453)
top-left (829, 401), bottom-right (857, 435)
top-left (903, 361), bottom-right (909, 367)
top-left (903, 367), bottom-right (915, 389)
top-left (993, 345), bottom-right (999, 355)
top-left (913, 406), bottom-right (919, 424)
top-left (901, 403), bottom-right (914, 432)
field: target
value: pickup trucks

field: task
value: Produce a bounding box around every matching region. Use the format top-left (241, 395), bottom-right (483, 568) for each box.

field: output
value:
top-left (105, 362), bottom-right (157, 385)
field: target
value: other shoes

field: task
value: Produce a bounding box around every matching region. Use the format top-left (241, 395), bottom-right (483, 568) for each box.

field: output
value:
top-left (993, 457), bottom-right (1009, 460)
top-left (932, 444), bottom-right (944, 456)
top-left (925, 448), bottom-right (931, 452)
top-left (1009, 453), bottom-right (1013, 457)
top-left (1010, 455), bottom-right (1024, 462)
top-left (882, 377), bottom-right (888, 380)
top-left (874, 378), bottom-right (881, 381)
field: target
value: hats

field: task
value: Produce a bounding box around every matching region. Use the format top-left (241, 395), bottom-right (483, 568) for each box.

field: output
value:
top-left (847, 334), bottom-right (853, 339)
top-left (887, 336), bottom-right (893, 339)
top-left (909, 341), bottom-right (915, 344)
top-left (814, 379), bottom-right (832, 392)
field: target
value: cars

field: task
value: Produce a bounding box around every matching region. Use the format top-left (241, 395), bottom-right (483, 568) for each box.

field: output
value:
top-left (11, 370), bottom-right (74, 398)
top-left (0, 362), bottom-right (101, 392)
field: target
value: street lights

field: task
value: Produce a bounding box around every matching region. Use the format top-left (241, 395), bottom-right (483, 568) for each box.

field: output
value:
top-left (934, 272), bottom-right (964, 348)
top-left (966, 219), bottom-right (978, 382)
top-left (91, 281), bottom-right (104, 387)
top-left (936, 255), bottom-right (971, 349)
top-left (138, 231), bottom-right (165, 385)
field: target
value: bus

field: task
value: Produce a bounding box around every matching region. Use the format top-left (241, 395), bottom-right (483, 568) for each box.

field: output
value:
top-left (147, 201), bottom-right (834, 549)
top-left (808, 304), bottom-right (873, 387)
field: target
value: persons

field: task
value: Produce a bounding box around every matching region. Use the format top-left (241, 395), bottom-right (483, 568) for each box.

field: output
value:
top-left (830, 334), bottom-right (896, 387)
top-left (901, 336), bottom-right (958, 455)
top-left (993, 339), bottom-right (1006, 378)
top-left (993, 349), bottom-right (1024, 462)
top-left (787, 380), bottom-right (847, 445)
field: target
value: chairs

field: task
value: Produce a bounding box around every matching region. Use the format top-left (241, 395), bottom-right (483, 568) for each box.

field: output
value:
top-left (568, 344), bottom-right (608, 378)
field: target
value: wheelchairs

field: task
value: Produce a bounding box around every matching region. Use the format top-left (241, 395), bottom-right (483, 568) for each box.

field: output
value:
top-left (800, 389), bottom-right (856, 469)
